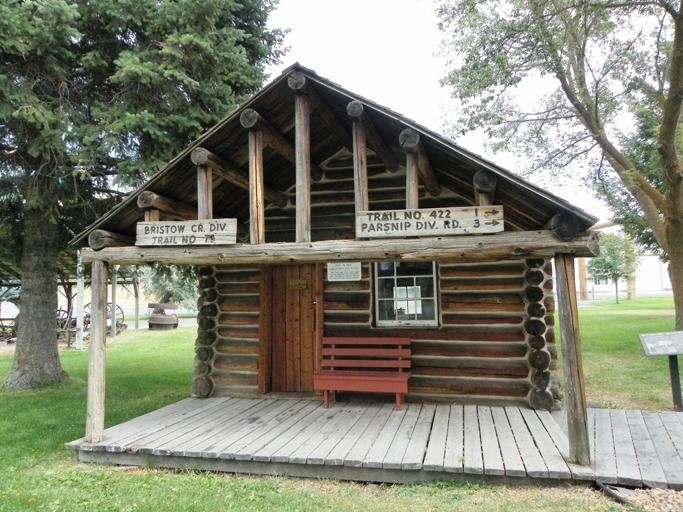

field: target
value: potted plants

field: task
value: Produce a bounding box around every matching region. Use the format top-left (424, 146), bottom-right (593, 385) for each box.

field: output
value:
top-left (397, 307), bottom-right (405, 314)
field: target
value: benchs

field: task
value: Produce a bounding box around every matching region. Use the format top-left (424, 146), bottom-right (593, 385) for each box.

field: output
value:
top-left (312, 335), bottom-right (410, 410)
top-left (0, 304), bottom-right (124, 348)
